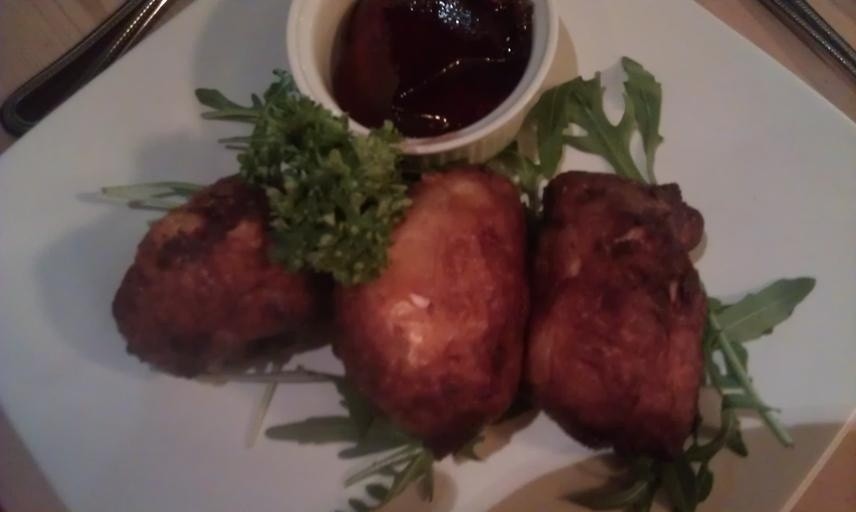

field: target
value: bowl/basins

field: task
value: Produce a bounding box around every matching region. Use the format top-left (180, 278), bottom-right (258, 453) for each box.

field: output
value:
top-left (283, 0), bottom-right (561, 169)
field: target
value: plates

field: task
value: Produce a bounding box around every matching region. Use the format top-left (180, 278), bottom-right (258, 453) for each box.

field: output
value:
top-left (0, 0), bottom-right (853, 512)
